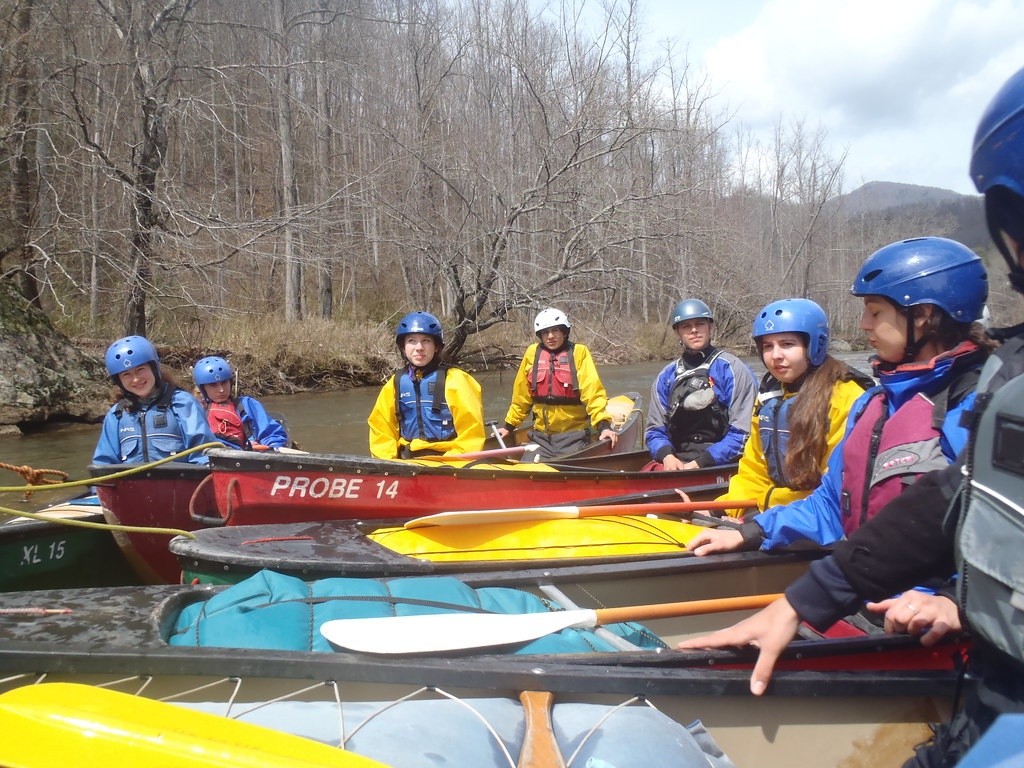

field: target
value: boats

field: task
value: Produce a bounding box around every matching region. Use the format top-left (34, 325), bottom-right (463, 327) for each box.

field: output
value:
top-left (0, 491), bottom-right (136, 593)
top-left (169, 482), bottom-right (729, 583)
top-left (188, 446), bottom-right (738, 527)
top-left (150, 549), bottom-right (967, 673)
top-left (86, 391), bottom-right (642, 584)
top-left (0, 645), bottom-right (960, 768)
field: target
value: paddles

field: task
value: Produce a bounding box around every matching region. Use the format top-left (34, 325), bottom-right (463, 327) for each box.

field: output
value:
top-left (2, 682), bottom-right (388, 768)
top-left (401, 498), bottom-right (757, 531)
top-left (418, 393), bottom-right (636, 462)
top-left (316, 589), bottom-right (781, 659)
top-left (252, 444), bottom-right (310, 455)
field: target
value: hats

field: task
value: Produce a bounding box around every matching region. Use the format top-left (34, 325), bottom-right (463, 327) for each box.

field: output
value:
top-left (671, 299), bottom-right (713, 328)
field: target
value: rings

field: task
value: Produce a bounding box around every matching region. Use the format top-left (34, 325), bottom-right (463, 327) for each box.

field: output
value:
top-left (907, 603), bottom-right (918, 613)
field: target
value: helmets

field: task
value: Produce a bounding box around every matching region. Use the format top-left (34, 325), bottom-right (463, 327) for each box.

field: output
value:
top-left (533, 309), bottom-right (570, 339)
top-left (969, 65), bottom-right (1024, 199)
top-left (753, 298), bottom-right (829, 365)
top-left (396, 312), bottom-right (445, 352)
top-left (193, 357), bottom-right (233, 401)
top-left (849, 237), bottom-right (989, 323)
top-left (105, 336), bottom-right (162, 393)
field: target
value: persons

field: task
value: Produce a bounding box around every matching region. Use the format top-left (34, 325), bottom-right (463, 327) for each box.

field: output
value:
top-left (677, 66), bottom-right (1024, 768)
top-left (675, 298), bottom-right (875, 542)
top-left (193, 356), bottom-right (287, 452)
top-left (367, 311), bottom-right (486, 468)
top-left (685, 236), bottom-right (998, 646)
top-left (491, 308), bottom-right (618, 463)
top-left (92, 335), bottom-right (220, 494)
top-left (644, 299), bottom-right (757, 471)
